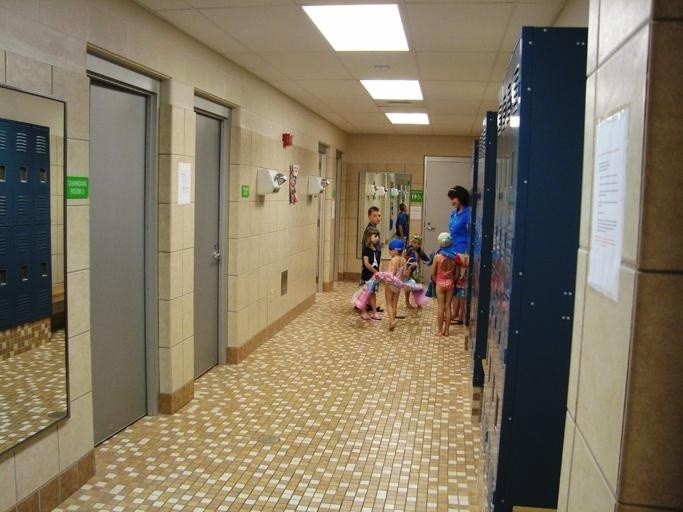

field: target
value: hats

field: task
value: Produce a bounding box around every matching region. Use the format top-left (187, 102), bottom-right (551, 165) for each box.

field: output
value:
top-left (410, 233), bottom-right (423, 243)
top-left (439, 232), bottom-right (451, 246)
top-left (389, 240), bottom-right (404, 251)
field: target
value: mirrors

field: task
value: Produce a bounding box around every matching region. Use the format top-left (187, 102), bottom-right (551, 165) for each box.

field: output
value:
top-left (357, 171), bottom-right (412, 260)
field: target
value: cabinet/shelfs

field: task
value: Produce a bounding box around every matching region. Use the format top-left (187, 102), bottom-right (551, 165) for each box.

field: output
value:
top-left (420, 155), bottom-right (472, 283)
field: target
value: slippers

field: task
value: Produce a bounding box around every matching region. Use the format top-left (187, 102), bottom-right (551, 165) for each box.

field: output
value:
top-left (354, 304), bottom-right (384, 320)
top-left (450, 318), bottom-right (463, 324)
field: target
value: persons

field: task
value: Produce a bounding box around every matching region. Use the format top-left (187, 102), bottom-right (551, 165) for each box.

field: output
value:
top-left (403, 234), bottom-right (430, 309)
top-left (394, 202), bottom-right (410, 245)
top-left (442, 183), bottom-right (469, 326)
top-left (427, 231), bottom-right (467, 338)
top-left (382, 238), bottom-right (408, 330)
top-left (361, 204), bottom-right (384, 313)
top-left (359, 226), bottom-right (381, 321)
